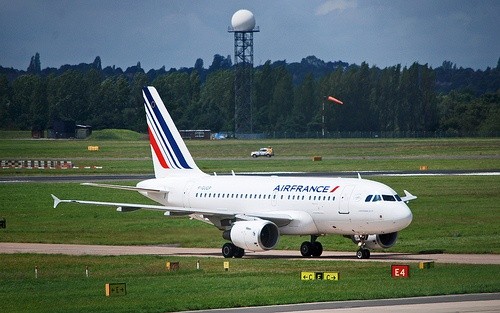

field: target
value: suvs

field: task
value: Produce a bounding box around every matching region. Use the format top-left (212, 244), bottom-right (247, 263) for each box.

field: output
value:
top-left (250, 147), bottom-right (274, 158)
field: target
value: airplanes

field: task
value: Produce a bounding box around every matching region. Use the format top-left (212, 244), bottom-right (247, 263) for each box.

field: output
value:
top-left (49, 84), bottom-right (418, 259)
